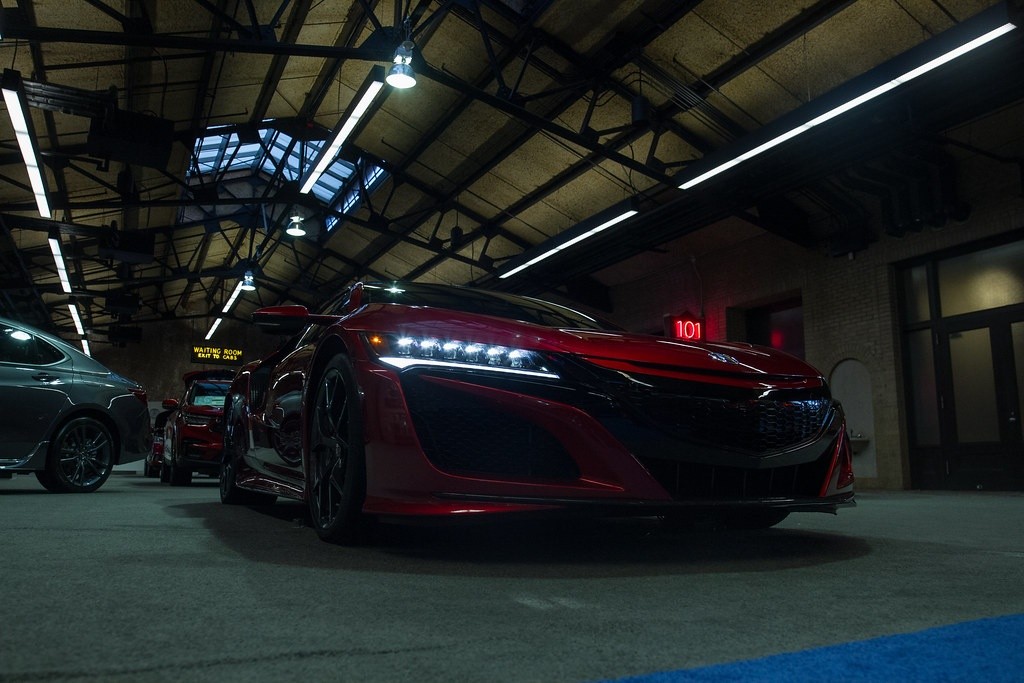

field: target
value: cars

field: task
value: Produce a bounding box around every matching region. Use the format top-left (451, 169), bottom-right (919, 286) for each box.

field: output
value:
top-left (0, 317), bottom-right (154, 493)
top-left (146, 282), bottom-right (859, 547)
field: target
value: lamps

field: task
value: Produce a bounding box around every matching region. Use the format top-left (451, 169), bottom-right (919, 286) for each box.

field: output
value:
top-left (286, 212), bottom-right (308, 237)
top-left (0, 27), bottom-right (53, 219)
top-left (451, 193), bottom-right (464, 249)
top-left (387, 15), bottom-right (417, 88)
top-left (241, 271), bottom-right (256, 291)
top-left (631, 66), bottom-right (650, 129)
top-left (669, 0), bottom-right (1020, 192)
top-left (67, 300), bottom-right (86, 335)
top-left (203, 316), bottom-right (223, 342)
top-left (298, 64), bottom-right (385, 194)
top-left (493, 195), bottom-right (642, 280)
top-left (220, 276), bottom-right (244, 312)
top-left (81, 336), bottom-right (93, 357)
top-left (47, 231), bottom-right (73, 294)
